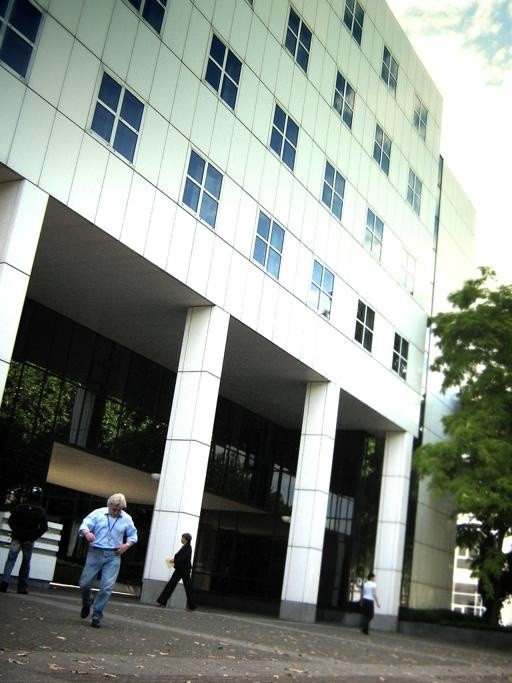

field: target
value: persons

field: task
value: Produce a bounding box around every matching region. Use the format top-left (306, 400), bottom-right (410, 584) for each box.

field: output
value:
top-left (76, 492), bottom-right (140, 629)
top-left (153, 531), bottom-right (200, 611)
top-left (1, 483), bottom-right (49, 594)
top-left (359, 572), bottom-right (381, 634)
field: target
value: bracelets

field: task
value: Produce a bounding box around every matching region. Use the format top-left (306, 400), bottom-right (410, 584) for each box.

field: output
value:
top-left (125, 542), bottom-right (132, 548)
top-left (83, 530), bottom-right (91, 536)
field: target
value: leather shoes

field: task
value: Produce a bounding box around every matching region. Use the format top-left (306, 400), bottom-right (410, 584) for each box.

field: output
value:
top-left (91, 619), bottom-right (100, 628)
top-left (81, 599), bottom-right (94, 618)
